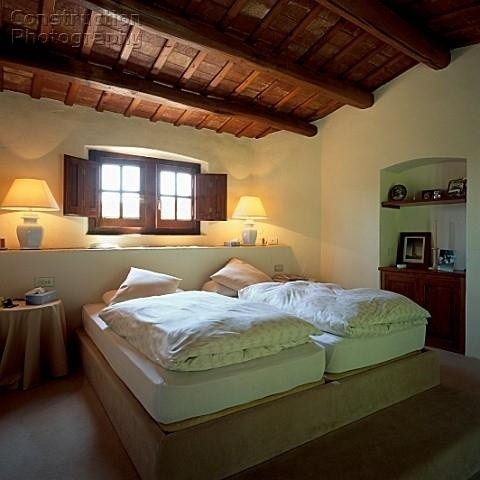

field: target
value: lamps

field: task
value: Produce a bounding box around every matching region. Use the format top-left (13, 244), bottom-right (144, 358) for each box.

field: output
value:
top-left (230, 194), bottom-right (267, 244)
top-left (1, 173), bottom-right (63, 252)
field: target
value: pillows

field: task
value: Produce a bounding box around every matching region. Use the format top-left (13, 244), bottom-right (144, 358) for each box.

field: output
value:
top-left (100, 286), bottom-right (118, 305)
top-left (210, 255), bottom-right (275, 292)
top-left (111, 263), bottom-right (183, 303)
top-left (199, 279), bottom-right (237, 299)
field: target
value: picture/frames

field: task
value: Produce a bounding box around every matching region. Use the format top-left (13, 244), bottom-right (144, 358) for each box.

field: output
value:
top-left (396, 230), bottom-right (433, 270)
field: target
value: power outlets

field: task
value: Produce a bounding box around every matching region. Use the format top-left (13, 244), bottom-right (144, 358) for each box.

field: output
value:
top-left (33, 276), bottom-right (55, 288)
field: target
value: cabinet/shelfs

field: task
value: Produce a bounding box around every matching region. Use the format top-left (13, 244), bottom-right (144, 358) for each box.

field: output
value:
top-left (377, 264), bottom-right (464, 356)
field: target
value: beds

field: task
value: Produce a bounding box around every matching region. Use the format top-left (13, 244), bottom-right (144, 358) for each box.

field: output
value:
top-left (203, 267), bottom-right (441, 442)
top-left (76, 286), bottom-right (330, 477)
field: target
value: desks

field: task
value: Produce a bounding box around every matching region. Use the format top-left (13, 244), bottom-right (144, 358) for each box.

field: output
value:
top-left (1, 296), bottom-right (72, 388)
